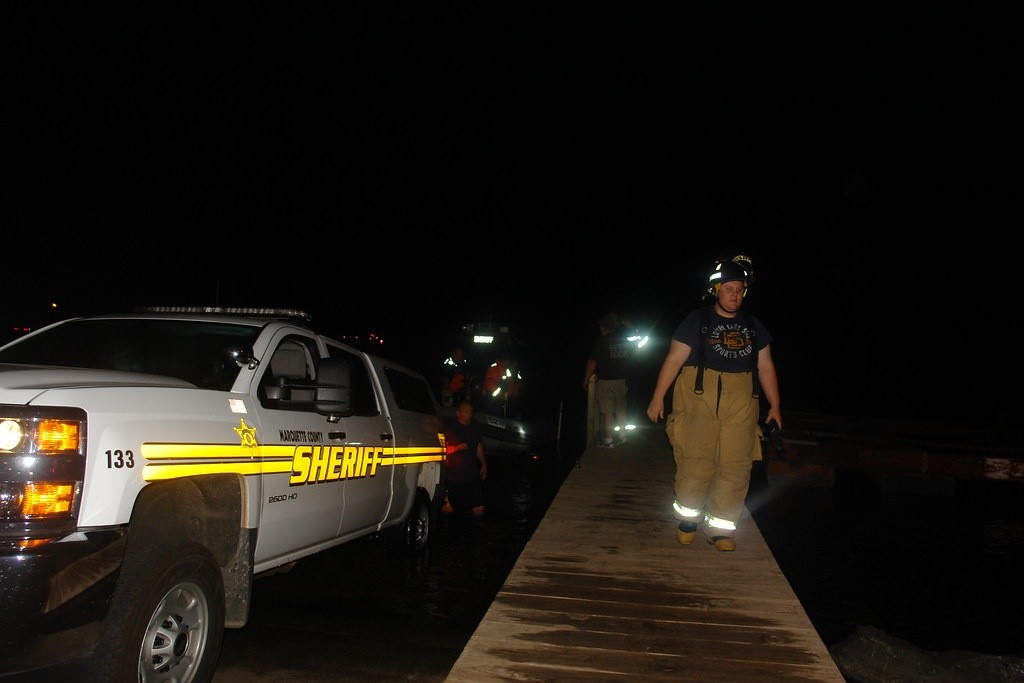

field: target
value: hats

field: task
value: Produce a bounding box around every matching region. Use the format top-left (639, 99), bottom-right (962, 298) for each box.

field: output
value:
top-left (598, 317), bottom-right (613, 325)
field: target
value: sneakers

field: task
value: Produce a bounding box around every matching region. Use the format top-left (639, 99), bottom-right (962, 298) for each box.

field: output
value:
top-left (592, 439), bottom-right (624, 449)
top-left (707, 536), bottom-right (736, 551)
top-left (677, 521), bottom-right (697, 544)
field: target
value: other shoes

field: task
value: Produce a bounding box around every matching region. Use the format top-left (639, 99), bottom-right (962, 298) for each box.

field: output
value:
top-left (472, 518), bottom-right (496, 529)
top-left (440, 520), bottom-right (458, 529)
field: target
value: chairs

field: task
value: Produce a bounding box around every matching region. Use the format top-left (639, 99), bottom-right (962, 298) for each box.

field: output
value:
top-left (266, 343), bottom-right (313, 401)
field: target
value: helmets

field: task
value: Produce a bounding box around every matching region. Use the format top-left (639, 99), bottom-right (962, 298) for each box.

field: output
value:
top-left (707, 255), bottom-right (753, 297)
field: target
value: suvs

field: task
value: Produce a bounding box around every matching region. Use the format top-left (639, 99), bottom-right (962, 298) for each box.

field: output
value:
top-left (1, 305), bottom-right (451, 683)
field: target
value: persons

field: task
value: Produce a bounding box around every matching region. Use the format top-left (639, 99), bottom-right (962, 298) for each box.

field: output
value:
top-left (437, 346), bottom-right (520, 519)
top-left (584, 313), bottom-right (634, 449)
top-left (647, 261), bottom-right (782, 550)
